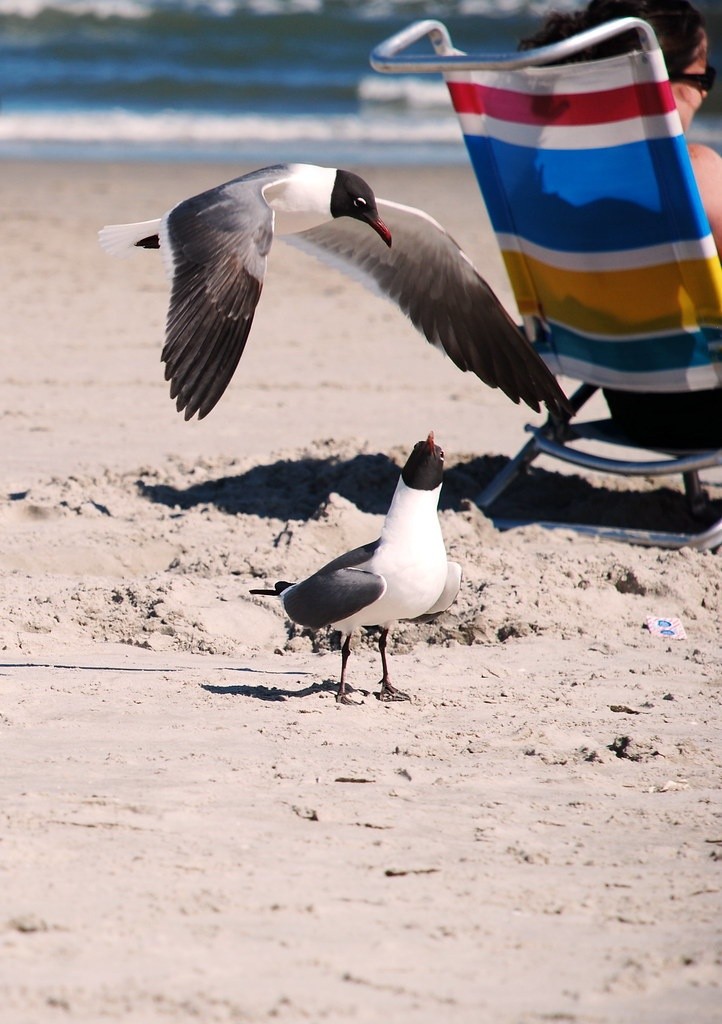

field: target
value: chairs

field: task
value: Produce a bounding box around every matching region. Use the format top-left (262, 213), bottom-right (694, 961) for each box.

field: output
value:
top-left (369, 17), bottom-right (721, 553)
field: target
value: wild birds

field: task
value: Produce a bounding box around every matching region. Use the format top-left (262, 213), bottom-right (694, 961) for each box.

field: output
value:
top-left (248, 431), bottom-right (461, 709)
top-left (98, 161), bottom-right (578, 422)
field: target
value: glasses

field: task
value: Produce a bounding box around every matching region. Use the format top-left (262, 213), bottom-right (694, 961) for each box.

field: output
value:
top-left (674, 65), bottom-right (716, 90)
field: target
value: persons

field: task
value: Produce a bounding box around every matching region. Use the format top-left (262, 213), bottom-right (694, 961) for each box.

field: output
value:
top-left (513, 0), bottom-right (721, 453)
